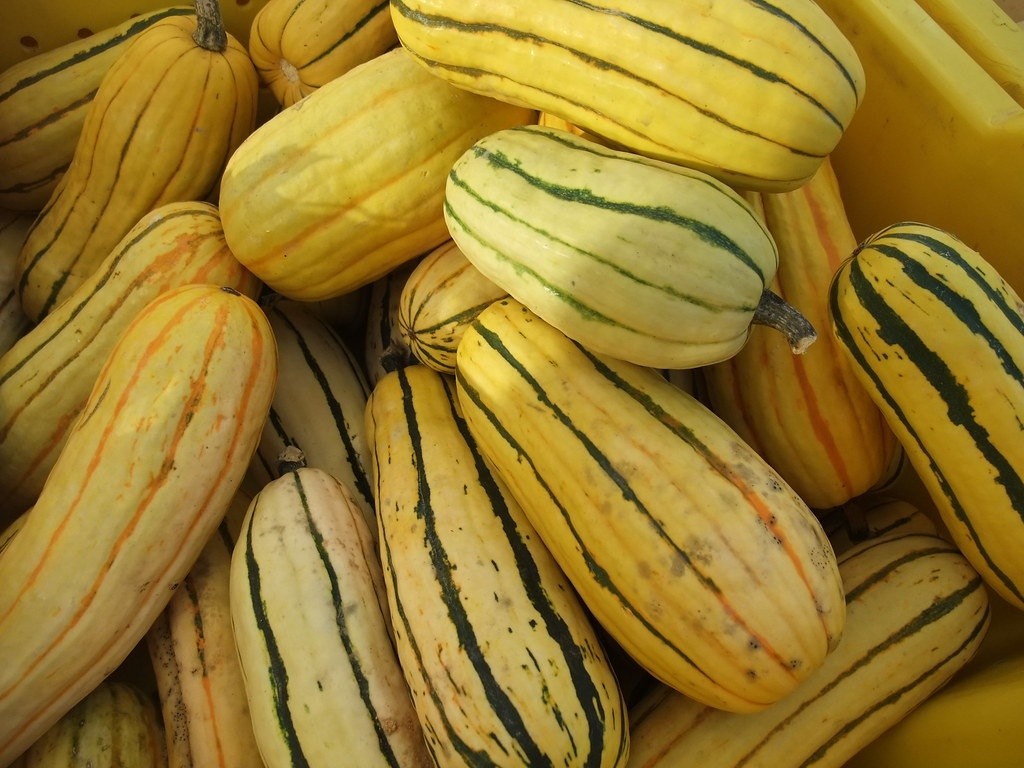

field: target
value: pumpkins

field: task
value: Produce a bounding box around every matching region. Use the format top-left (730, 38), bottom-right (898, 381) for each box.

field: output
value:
top-left (0, 0), bottom-right (1024, 768)
